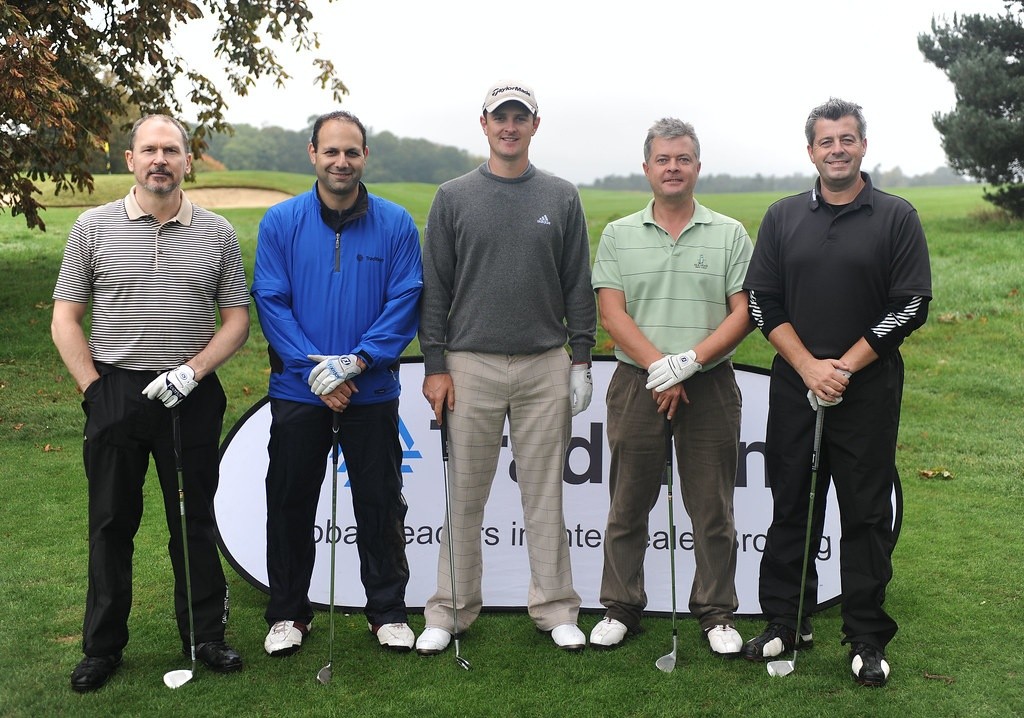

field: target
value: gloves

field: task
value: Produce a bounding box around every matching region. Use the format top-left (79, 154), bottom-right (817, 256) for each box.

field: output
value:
top-left (142, 364), bottom-right (199, 410)
top-left (568, 363), bottom-right (593, 414)
top-left (307, 352), bottom-right (362, 396)
top-left (807, 368), bottom-right (852, 411)
top-left (645, 349), bottom-right (702, 392)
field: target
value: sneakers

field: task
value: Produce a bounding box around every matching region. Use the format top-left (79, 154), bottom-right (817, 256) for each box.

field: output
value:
top-left (588, 615), bottom-right (628, 649)
top-left (703, 623), bottom-right (743, 659)
top-left (264, 620), bottom-right (311, 656)
top-left (742, 623), bottom-right (813, 660)
top-left (849, 641), bottom-right (891, 687)
top-left (71, 650), bottom-right (123, 692)
top-left (181, 640), bottom-right (243, 673)
top-left (415, 626), bottom-right (451, 654)
top-left (552, 624), bottom-right (587, 650)
top-left (366, 619), bottom-right (416, 652)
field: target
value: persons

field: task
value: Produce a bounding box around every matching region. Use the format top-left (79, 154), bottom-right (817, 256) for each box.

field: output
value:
top-left (742, 98), bottom-right (932, 687)
top-left (417, 79), bottom-right (594, 657)
top-left (254, 111), bottom-right (421, 655)
top-left (587, 118), bottom-right (757, 655)
top-left (49, 115), bottom-right (252, 694)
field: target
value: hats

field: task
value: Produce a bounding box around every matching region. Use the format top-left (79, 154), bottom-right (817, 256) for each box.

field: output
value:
top-left (482, 79), bottom-right (538, 119)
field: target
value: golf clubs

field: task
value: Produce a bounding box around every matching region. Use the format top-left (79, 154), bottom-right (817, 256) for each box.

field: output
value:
top-left (317, 410), bottom-right (343, 686)
top-left (163, 409), bottom-right (198, 690)
top-left (438, 400), bottom-right (473, 670)
top-left (766, 403), bottom-right (828, 679)
top-left (654, 408), bottom-right (679, 674)
